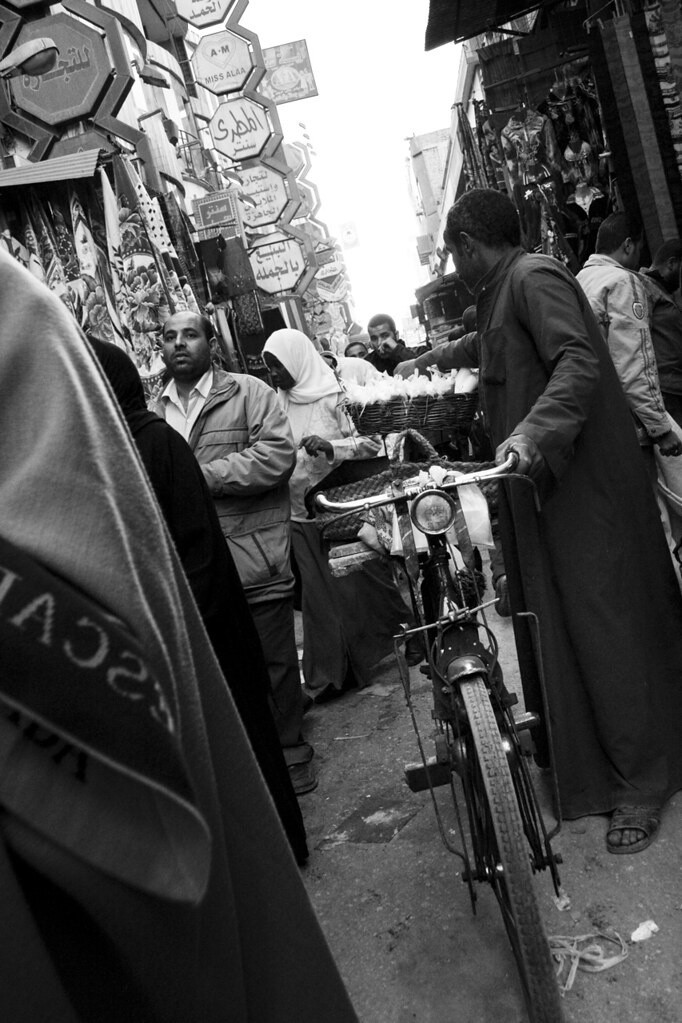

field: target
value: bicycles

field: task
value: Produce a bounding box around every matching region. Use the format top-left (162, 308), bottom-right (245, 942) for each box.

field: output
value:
top-left (305, 448), bottom-right (572, 1023)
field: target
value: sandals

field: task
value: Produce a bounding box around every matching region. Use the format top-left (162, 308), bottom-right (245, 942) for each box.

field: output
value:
top-left (607, 802), bottom-right (663, 854)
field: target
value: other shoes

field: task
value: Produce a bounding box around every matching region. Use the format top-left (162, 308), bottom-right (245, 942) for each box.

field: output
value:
top-left (288, 760), bottom-right (319, 796)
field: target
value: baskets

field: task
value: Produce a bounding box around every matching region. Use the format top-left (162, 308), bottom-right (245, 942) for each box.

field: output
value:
top-left (311, 428), bottom-right (503, 541)
top-left (345, 391), bottom-right (479, 431)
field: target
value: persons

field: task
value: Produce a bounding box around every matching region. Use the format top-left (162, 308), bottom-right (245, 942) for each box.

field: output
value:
top-left (556, 194), bottom-right (617, 273)
top-left (3, 229), bottom-right (361, 1023)
top-left (82, 334), bottom-right (314, 871)
top-left (630, 236), bottom-right (681, 433)
top-left (319, 305), bottom-right (523, 623)
top-left (258, 326), bottom-right (430, 714)
top-left (143, 310), bottom-right (322, 803)
top-left (388, 186), bottom-right (682, 855)
top-left (573, 210), bottom-right (682, 596)
